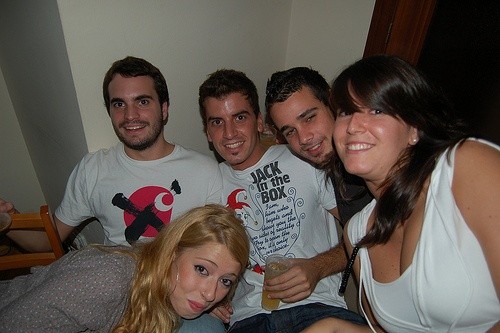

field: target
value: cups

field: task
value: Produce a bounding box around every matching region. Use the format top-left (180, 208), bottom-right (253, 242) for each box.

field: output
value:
top-left (0, 212), bottom-right (12, 255)
top-left (262, 254), bottom-right (292, 311)
top-left (259, 126), bottom-right (277, 148)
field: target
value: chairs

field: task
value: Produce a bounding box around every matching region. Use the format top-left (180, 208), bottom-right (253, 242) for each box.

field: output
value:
top-left (0, 205), bottom-right (64, 269)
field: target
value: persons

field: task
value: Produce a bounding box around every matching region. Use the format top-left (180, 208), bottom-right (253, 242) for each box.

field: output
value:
top-left (318, 56), bottom-right (500, 333)
top-left (0, 56), bottom-right (225, 333)
top-left (199, 68), bottom-right (368, 333)
top-left (263, 67), bottom-right (375, 227)
top-left (0, 205), bottom-right (250, 333)
